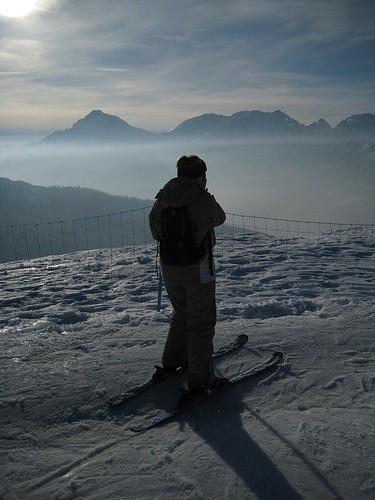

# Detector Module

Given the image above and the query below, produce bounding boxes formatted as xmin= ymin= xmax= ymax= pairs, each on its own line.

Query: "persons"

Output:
xmin=149 ymin=155 xmax=229 ymax=390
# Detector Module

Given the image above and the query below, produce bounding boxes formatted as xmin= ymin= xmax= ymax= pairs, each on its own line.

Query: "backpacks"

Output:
xmin=160 ymin=187 xmax=213 ymax=275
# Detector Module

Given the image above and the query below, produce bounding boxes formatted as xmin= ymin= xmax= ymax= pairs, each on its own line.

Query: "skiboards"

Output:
xmin=105 ymin=333 xmax=284 ymax=433
